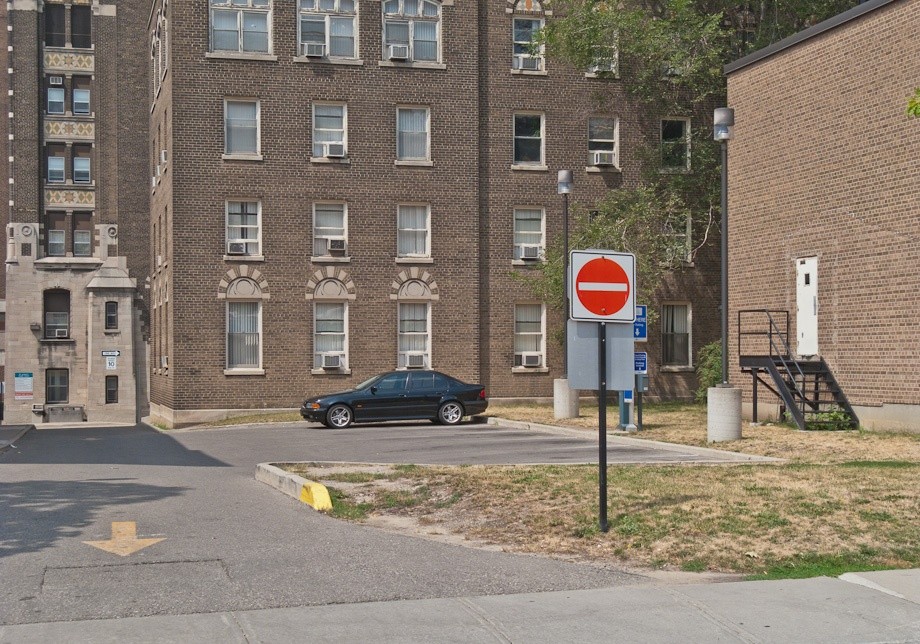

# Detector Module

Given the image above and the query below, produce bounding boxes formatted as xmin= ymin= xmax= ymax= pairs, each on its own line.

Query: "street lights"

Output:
xmin=553 ymin=168 xmax=581 ymax=421
xmin=707 ymin=108 xmax=744 ymax=440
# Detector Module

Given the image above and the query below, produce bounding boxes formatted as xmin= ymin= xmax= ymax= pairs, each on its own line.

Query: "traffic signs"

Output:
xmin=102 ymin=350 xmax=120 ymax=356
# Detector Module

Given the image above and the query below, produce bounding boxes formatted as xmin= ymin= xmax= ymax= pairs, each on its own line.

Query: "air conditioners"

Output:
xmin=405 ymin=353 xmax=425 ymax=367
xmin=387 ymin=45 xmax=409 ymax=60
xmin=594 ymin=57 xmax=612 ymax=72
xmin=522 ymin=352 xmax=541 ymax=366
xmin=301 ymin=43 xmax=325 ymax=56
xmin=323 ymin=142 xmax=345 ymax=158
xmin=588 ymin=151 xmax=615 ymax=166
xmin=327 ymin=239 xmax=345 ymax=251
xmin=518 ymin=56 xmax=539 ymax=70
xmin=56 ymin=329 xmax=67 ymax=337
xmin=518 ymin=246 xmax=539 ymax=258
xmin=322 ymin=354 xmax=341 ymax=367
xmin=228 ymin=242 xmax=247 ymax=253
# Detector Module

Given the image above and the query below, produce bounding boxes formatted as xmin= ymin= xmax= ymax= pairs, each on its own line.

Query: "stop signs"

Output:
xmin=576 ymin=258 xmax=629 ymax=315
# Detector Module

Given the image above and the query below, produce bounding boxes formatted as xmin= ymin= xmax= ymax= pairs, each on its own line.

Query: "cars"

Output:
xmin=301 ymin=370 xmax=488 ymax=429
